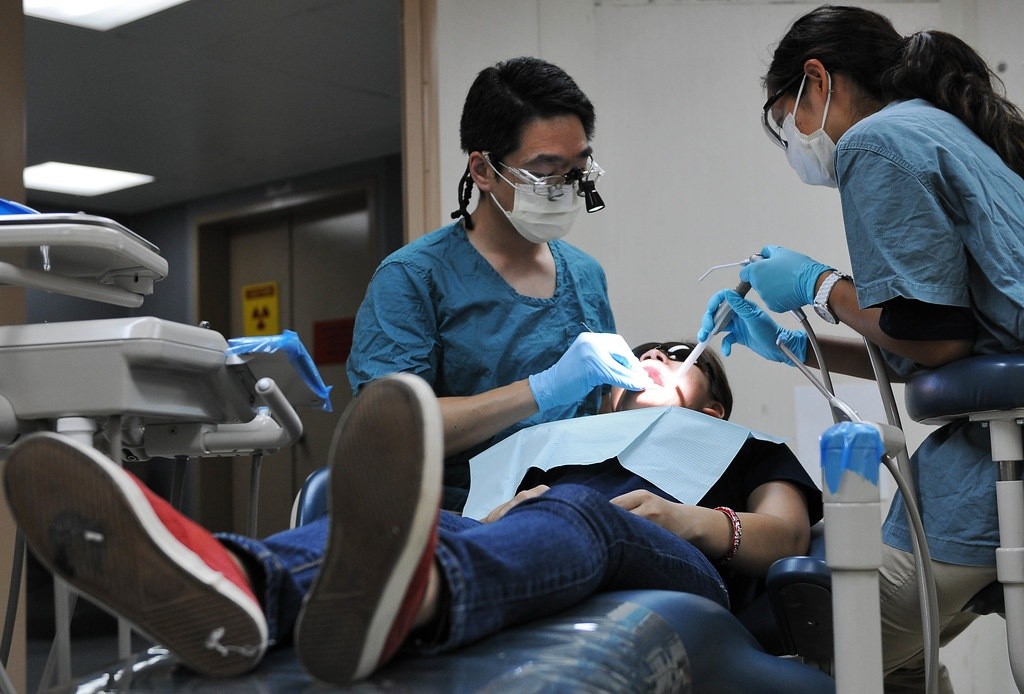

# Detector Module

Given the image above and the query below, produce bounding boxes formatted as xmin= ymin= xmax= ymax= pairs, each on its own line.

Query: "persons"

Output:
xmin=698 ymin=4 xmax=1024 ymax=694
xmin=345 ymin=59 xmax=652 ymax=497
xmin=0 ymin=343 xmax=826 ymax=683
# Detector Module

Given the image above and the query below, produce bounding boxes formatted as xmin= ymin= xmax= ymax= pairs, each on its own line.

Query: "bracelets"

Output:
xmin=711 ymin=507 xmax=741 ymax=569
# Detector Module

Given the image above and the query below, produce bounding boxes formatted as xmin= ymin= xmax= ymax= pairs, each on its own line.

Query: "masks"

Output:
xmin=480 ymin=150 xmax=586 ymax=245
xmin=776 ymin=70 xmax=839 ymax=189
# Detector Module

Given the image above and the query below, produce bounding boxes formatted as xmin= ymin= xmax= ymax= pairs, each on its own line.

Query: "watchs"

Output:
xmin=813 ymin=270 xmax=854 ymax=325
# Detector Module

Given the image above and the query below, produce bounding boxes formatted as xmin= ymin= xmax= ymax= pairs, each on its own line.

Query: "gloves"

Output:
xmin=697 ymin=289 xmax=806 ymax=367
xmin=739 ymin=245 xmax=839 ymax=314
xmin=528 ymin=332 xmax=653 ymax=412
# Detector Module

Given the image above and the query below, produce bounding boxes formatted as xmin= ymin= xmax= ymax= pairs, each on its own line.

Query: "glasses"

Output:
xmin=492 ymin=156 xmax=605 ymax=198
xmin=759 ymin=70 xmax=806 ymax=152
xmin=631 ymin=341 xmax=712 ymax=372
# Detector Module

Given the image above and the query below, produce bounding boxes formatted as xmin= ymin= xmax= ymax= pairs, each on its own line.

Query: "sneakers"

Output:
xmin=0 ymin=431 xmax=270 ymax=681
xmin=293 ymin=369 xmax=445 ymax=685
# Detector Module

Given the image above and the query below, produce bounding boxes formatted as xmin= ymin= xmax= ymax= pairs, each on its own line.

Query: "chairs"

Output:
xmin=57 ymin=465 xmax=836 ymax=694
